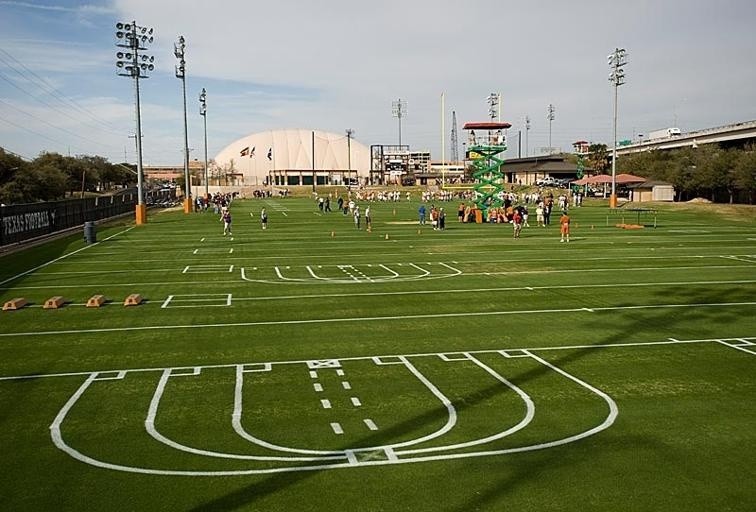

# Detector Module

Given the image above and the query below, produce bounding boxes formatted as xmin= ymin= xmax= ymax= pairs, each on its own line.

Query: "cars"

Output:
xmin=587 ymin=185 xmax=601 ymax=193
xmin=536 ymin=177 xmax=575 ymax=188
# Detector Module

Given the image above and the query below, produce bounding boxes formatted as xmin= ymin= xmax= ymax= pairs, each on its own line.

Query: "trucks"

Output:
xmin=648 ymin=126 xmax=681 ymax=142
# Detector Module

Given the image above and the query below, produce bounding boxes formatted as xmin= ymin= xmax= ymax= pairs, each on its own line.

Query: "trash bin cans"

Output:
xmin=83 ymin=221 xmax=97 ymax=246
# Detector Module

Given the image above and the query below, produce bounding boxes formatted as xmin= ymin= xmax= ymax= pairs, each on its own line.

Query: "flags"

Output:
xmin=249 ymin=147 xmax=255 ymax=159
xmin=239 ymin=147 xmax=248 ymax=157
xmin=267 ymin=147 xmax=271 ymax=160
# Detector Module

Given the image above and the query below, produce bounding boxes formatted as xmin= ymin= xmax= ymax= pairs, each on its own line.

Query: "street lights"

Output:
xmin=173 ymin=35 xmax=210 ymax=213
xmin=485 ymin=92 xmax=498 ymax=191
xmin=344 ymin=128 xmax=355 ymax=183
xmin=113 ymin=20 xmax=155 ymax=225
xmin=391 ymin=97 xmax=409 ymax=151
xmin=546 ymin=103 xmax=555 ymax=154
xmin=606 ymin=46 xmax=628 ymax=208
